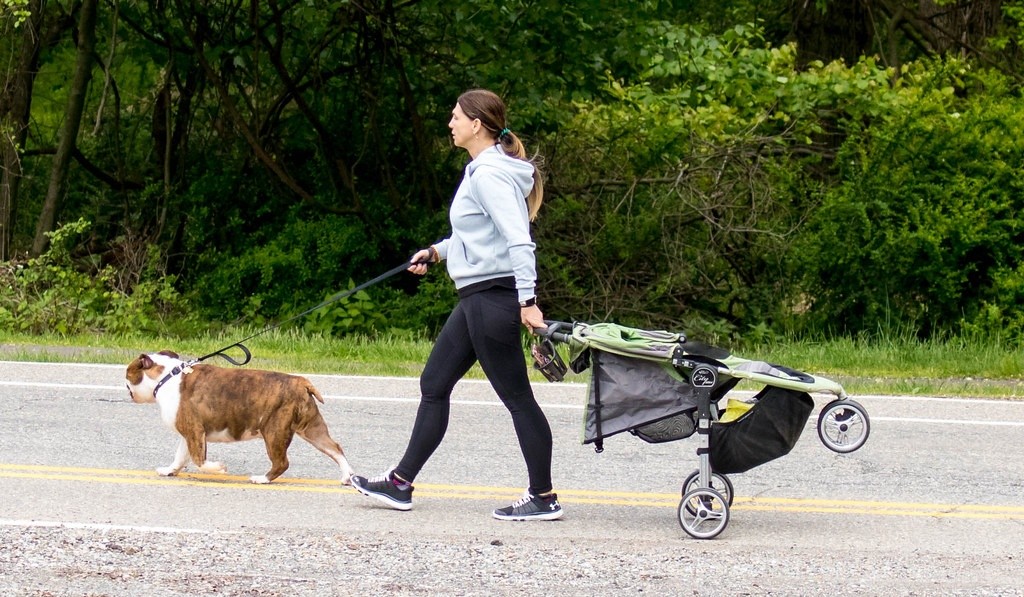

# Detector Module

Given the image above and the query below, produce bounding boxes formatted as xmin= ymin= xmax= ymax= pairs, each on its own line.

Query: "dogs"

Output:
xmin=124 ymin=349 xmax=359 ymax=485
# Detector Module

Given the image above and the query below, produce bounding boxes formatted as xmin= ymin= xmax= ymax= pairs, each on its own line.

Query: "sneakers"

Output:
xmin=350 ymin=470 xmax=415 ymax=510
xmin=492 ymin=486 xmax=564 ymax=521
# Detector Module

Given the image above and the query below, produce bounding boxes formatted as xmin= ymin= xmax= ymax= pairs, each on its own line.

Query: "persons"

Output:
xmin=350 ymin=90 xmax=564 ymax=521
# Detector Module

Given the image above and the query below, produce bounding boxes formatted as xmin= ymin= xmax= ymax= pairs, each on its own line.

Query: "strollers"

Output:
xmin=533 ymin=319 xmax=872 ymax=540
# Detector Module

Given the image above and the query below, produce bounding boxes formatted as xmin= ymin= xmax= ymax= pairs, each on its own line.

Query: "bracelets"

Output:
xmin=431 ymin=247 xmax=441 ymax=264
xmin=519 ymin=296 xmax=536 ymax=307
